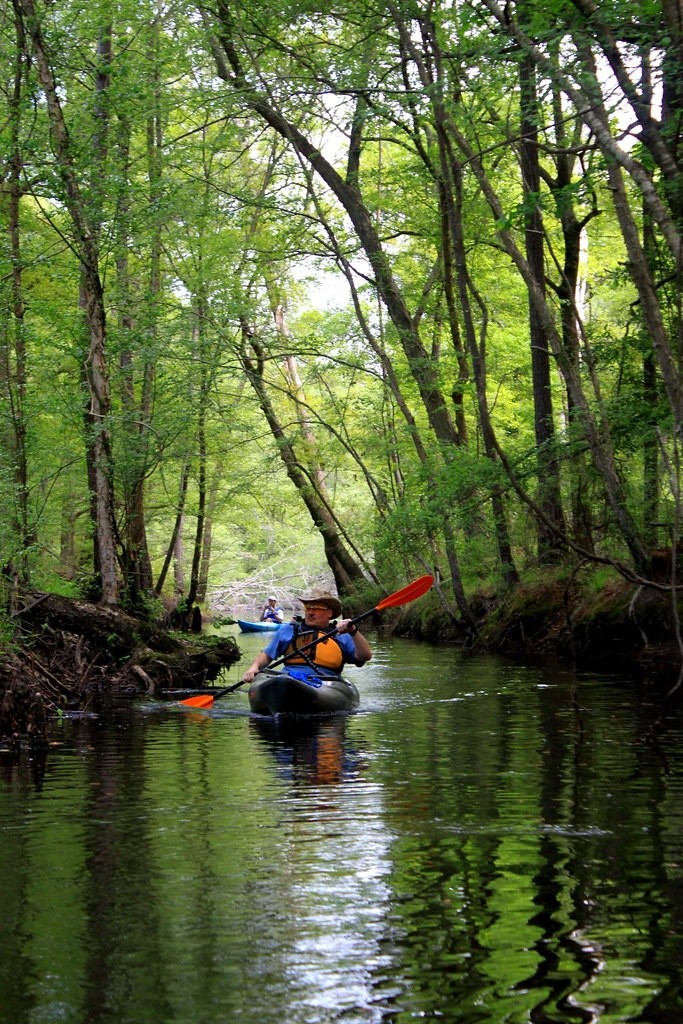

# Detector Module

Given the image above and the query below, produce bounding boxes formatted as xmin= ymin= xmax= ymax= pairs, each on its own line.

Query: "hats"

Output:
xmin=268 ymin=595 xmax=276 ymax=600
xmin=297 ymin=585 xmax=342 ymax=619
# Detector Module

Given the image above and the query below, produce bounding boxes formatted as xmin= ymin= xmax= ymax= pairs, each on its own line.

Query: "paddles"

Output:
xmin=179 ymin=574 xmax=434 ymax=710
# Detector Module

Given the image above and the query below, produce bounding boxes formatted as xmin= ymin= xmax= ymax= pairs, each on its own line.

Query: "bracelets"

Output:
xmin=350 ymin=627 xmax=358 ymax=636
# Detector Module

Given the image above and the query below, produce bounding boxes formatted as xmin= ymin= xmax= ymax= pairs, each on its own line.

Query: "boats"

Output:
xmin=248 ymin=669 xmax=361 ymax=717
xmin=237 ymin=618 xmax=288 ymax=633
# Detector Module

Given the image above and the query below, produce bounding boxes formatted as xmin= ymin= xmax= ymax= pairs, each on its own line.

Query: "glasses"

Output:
xmin=303 ymin=603 xmax=328 ymax=612
xmin=269 ymin=600 xmax=274 ymax=602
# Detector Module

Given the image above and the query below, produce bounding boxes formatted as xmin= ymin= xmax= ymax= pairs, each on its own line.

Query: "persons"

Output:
xmin=243 ymin=589 xmax=372 ymax=683
xmin=260 ymin=596 xmax=283 ymax=624
